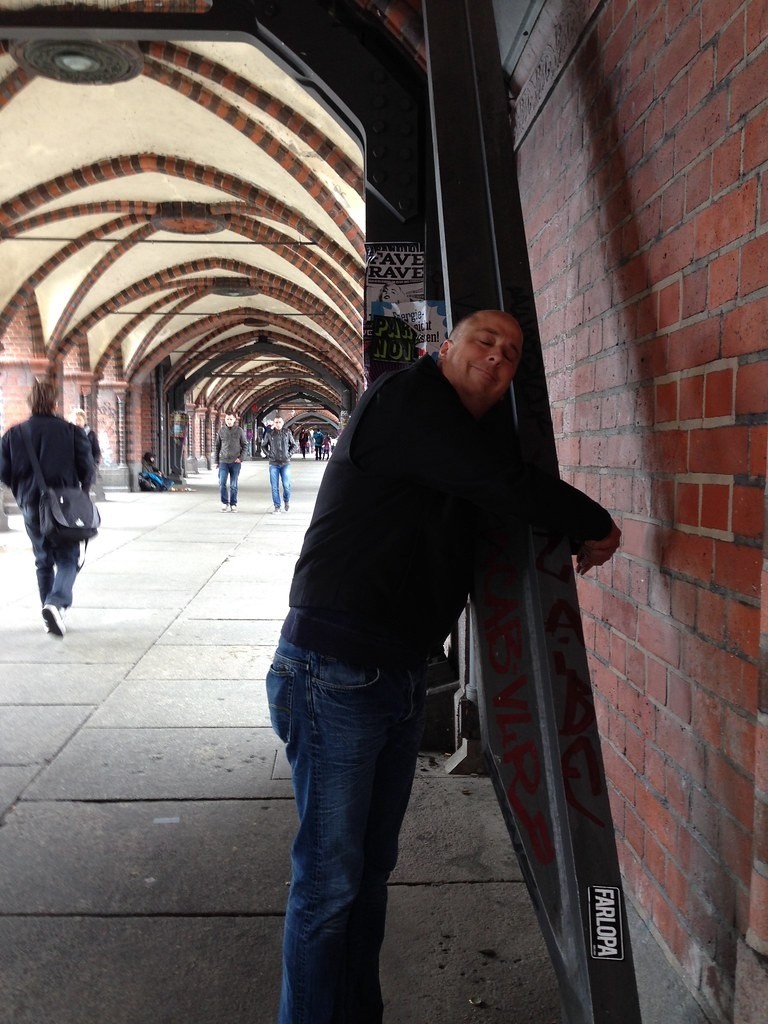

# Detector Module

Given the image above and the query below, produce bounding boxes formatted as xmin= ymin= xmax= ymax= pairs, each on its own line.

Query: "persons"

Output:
xmin=142 ymin=452 xmax=174 ymax=489
xmin=261 ymin=417 xmax=296 ymax=513
xmin=266 ymin=310 xmax=620 ymax=1024
xmin=0 ymin=382 xmax=100 ymax=638
xmin=214 ymin=412 xmax=247 ymax=513
xmin=300 ymin=428 xmax=336 ymax=461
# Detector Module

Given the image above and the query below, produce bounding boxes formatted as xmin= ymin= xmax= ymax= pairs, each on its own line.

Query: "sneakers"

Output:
xmin=43 ymin=605 xmax=65 ymax=636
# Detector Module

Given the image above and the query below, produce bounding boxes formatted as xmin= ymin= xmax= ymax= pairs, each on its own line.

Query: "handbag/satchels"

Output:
xmin=39 ymin=487 xmax=101 ymax=541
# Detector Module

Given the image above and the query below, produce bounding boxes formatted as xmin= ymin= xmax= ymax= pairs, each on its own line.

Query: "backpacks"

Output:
xmin=316 ymin=435 xmax=322 ymax=445
xmin=138 ymin=473 xmax=164 ymax=491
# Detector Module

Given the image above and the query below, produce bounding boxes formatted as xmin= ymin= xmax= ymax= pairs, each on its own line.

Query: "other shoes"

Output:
xmin=285 ymin=501 xmax=290 ymax=511
xmin=222 ymin=503 xmax=229 ymax=512
xmin=168 ymin=486 xmax=177 ymax=492
xmin=231 ymin=505 xmax=238 ymax=512
xmin=273 ymin=506 xmax=281 ymax=515
